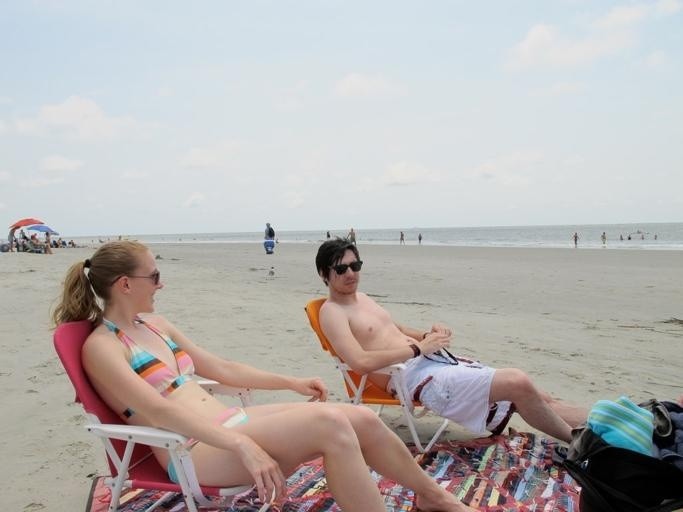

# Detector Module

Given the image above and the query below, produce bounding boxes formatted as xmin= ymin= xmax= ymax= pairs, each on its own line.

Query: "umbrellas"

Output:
xmin=8 ymin=217 xmax=45 ymax=230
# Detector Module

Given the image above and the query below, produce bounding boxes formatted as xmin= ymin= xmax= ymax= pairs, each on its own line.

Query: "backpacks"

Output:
xmin=551 ymin=398 xmax=682 ymax=512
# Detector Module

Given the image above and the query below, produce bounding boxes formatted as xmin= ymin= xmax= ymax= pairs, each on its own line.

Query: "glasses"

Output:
xmin=327 ymin=261 xmax=362 ymax=275
xmin=110 ymin=271 xmax=160 ymax=286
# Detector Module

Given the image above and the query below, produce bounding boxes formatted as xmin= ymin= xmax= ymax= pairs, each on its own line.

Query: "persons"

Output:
xmin=48 ymin=238 xmax=483 ymax=512
xmin=399 ymin=231 xmax=406 ymax=246
xmin=4 ymin=224 xmax=78 ymax=254
xmin=569 ymin=232 xmax=580 ymax=248
xmin=325 ymin=231 xmax=330 ymax=238
xmin=313 ymin=236 xmax=592 ymax=445
xmin=600 ymin=231 xmax=606 ymax=245
xmin=345 ymin=228 xmax=355 ymax=246
xmin=263 ymin=222 xmax=275 ymax=240
xmin=417 ymin=233 xmax=422 ymax=244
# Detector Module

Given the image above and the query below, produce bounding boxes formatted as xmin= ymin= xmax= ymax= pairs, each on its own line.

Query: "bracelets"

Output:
xmin=421 ymin=331 xmax=431 ymax=340
xmin=409 ymin=342 xmax=421 ymax=357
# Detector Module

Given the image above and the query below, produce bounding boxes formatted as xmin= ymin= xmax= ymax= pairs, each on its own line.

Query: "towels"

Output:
xmin=585 ymin=395 xmax=660 ymax=458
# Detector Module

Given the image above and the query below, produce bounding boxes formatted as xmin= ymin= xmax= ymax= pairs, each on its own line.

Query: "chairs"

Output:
xmin=305 ymin=297 xmax=449 ymax=453
xmin=53 ymin=318 xmax=275 ymax=511
